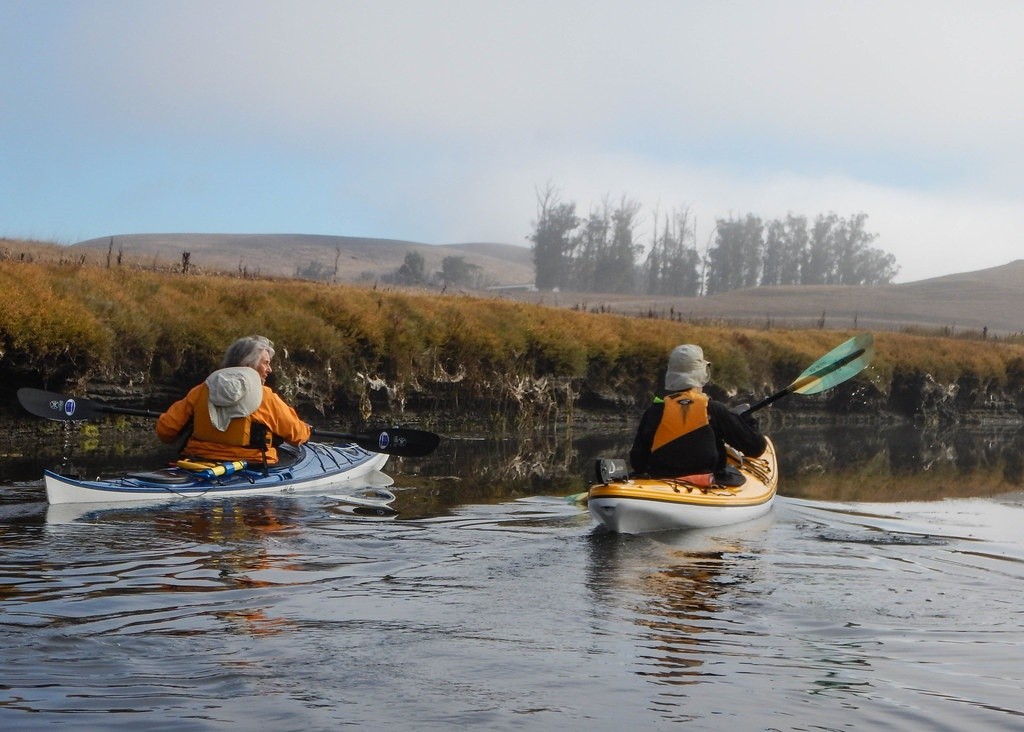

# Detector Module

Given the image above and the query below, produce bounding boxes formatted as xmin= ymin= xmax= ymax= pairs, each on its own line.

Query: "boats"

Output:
xmin=43 ymin=438 xmax=390 ymax=507
xmin=581 ymin=434 xmax=779 ymax=537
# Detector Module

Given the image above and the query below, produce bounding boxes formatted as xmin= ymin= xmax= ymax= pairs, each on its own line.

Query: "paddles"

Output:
xmin=17 ymin=387 xmax=441 ymax=459
xmin=575 ymin=330 xmax=876 ymax=501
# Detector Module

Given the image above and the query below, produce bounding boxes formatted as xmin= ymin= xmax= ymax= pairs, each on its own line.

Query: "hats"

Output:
xmin=205 ymin=366 xmax=263 ymax=431
xmin=665 ymin=343 xmax=711 ymax=391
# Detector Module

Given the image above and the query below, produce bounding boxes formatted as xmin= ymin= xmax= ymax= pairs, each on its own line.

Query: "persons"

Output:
xmin=156 ymin=335 xmax=311 ymax=465
xmin=629 ymin=345 xmax=768 ymax=484
